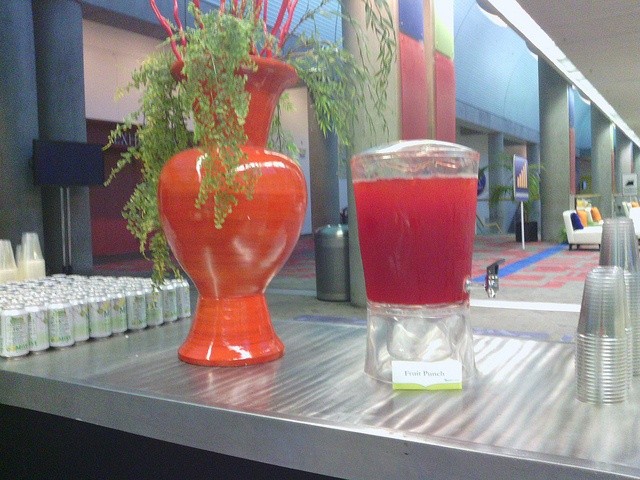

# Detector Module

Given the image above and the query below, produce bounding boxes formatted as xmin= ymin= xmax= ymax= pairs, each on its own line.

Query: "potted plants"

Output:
xmin=491 ymin=154 xmax=542 ymax=242
xmin=103 ymin=0 xmax=398 ymax=367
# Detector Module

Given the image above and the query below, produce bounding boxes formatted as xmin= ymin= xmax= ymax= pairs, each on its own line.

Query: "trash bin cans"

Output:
xmin=315 ymin=224 xmax=349 ymax=302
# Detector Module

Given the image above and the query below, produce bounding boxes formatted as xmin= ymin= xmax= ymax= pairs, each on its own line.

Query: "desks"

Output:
xmin=0 ymin=322 xmax=639 ymax=479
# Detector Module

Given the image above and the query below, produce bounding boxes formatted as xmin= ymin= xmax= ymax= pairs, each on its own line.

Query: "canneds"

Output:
xmin=0 ymin=273 xmax=190 ymax=358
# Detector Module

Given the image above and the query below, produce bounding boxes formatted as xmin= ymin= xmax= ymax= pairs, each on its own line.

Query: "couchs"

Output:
xmin=563 ymin=207 xmax=604 ymax=250
xmin=622 ymin=202 xmax=640 ymax=239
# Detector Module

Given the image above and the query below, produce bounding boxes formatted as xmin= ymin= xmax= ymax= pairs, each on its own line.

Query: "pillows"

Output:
xmin=571 ymin=213 xmax=583 ymax=229
xmin=627 ymin=203 xmax=632 ymax=212
xmin=592 ymin=207 xmax=601 ymax=221
xmin=577 ymin=211 xmax=587 ymax=226
xmin=586 ymin=213 xmax=593 ymax=226
xmin=632 ymin=202 xmax=639 ymax=207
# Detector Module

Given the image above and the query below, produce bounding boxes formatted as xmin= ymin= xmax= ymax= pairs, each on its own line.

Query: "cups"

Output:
xmin=351 ymin=139 xmax=480 ymax=385
xmin=0 ymin=231 xmax=47 ymax=285
xmin=575 ymin=266 xmax=633 ymax=405
xmin=598 ymin=216 xmax=640 ymax=377
